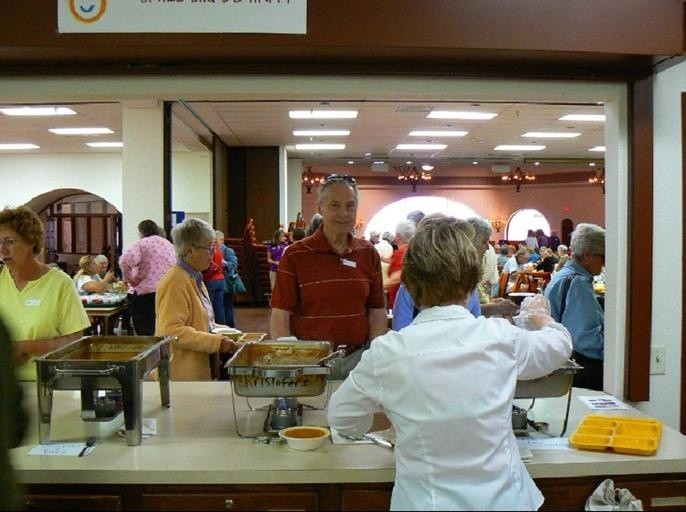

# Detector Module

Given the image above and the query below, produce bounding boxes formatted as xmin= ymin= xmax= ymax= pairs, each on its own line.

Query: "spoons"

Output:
xmin=79 ymin=436 xmax=96 ymax=456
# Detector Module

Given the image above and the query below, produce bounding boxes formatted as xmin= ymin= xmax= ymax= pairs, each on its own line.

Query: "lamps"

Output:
xmin=502 ymin=167 xmax=536 ymax=193
xmin=492 ymin=219 xmax=506 ymax=233
xmin=301 ymin=166 xmax=328 ymax=194
xmin=585 ymin=167 xmax=605 ymax=193
xmin=398 ymin=163 xmax=433 ymax=193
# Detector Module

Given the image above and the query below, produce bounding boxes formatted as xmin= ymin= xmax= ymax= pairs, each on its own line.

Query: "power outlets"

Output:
xmin=651 ymin=346 xmax=667 ymax=374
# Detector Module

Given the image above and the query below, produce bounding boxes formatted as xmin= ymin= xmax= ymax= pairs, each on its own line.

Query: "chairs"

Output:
xmin=497 ymin=273 xmax=510 ymax=299
xmin=223 ymin=217 xmax=278 ymax=308
xmin=528 ymin=271 xmax=551 ymax=293
xmin=512 ymin=273 xmax=524 ymax=293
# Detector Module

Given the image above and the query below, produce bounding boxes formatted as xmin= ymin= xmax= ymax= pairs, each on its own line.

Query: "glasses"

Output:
xmin=0 ymin=239 xmax=23 ymax=246
xmin=324 ymin=173 xmax=357 ymax=185
xmin=199 ymin=243 xmax=213 ymax=256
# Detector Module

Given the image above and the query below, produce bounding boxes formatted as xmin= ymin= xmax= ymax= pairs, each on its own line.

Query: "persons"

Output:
xmin=265 ymin=175 xmax=606 ymax=392
xmin=72 ymin=220 xmax=239 ymax=382
xmin=0 ymin=205 xmax=91 ymax=381
xmin=325 ymin=224 xmax=573 ymax=511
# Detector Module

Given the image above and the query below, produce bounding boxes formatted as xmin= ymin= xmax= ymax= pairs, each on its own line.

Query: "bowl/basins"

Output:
xmin=278 ymin=425 xmax=331 ymax=450
xmin=212 ymin=328 xmax=241 ymax=341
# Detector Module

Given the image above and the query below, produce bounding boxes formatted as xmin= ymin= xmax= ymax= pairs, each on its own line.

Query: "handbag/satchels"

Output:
xmin=230 ymin=273 xmax=247 ymax=295
xmin=569 ymin=352 xmax=606 ymax=392
